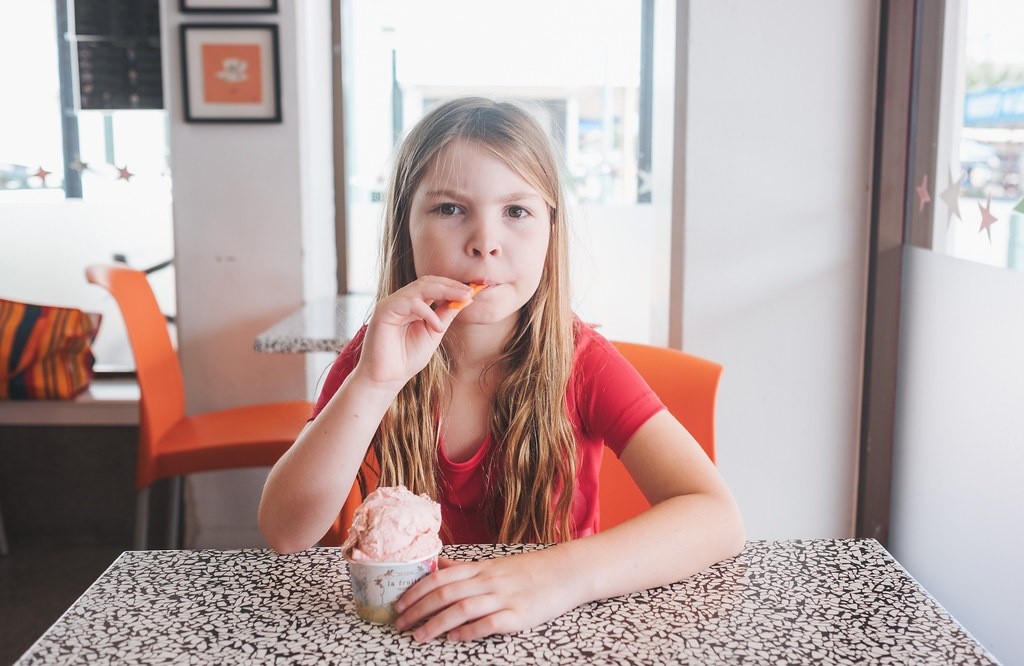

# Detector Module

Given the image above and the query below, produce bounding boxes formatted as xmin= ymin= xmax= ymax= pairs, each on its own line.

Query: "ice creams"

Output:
xmin=342 ymin=486 xmax=442 ymax=624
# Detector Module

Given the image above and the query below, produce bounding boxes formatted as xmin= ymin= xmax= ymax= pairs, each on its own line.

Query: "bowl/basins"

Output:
xmin=346 ymin=538 xmax=443 ymax=625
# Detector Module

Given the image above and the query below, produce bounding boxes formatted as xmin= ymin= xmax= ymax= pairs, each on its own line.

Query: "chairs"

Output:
xmin=83 ymin=263 xmax=314 ymax=551
xmin=595 ymin=341 xmax=722 ymax=532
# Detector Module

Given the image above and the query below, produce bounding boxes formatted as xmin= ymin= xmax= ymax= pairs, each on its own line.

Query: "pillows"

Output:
xmin=0 ymin=299 xmax=102 ymax=401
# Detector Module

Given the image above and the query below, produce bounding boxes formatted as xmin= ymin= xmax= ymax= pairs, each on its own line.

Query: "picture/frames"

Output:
xmin=179 ymin=0 xmax=278 ymax=13
xmin=179 ymin=23 xmax=283 ymax=124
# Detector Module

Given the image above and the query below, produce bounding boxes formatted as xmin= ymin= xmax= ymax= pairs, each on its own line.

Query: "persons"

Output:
xmin=260 ymin=98 xmax=747 ymax=644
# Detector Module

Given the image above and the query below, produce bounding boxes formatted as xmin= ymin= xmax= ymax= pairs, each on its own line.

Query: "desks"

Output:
xmin=250 ymin=293 xmax=378 ymax=353
xmin=10 ymin=538 xmax=1004 ymax=666
xmin=1 ymin=376 xmax=143 ymax=613
